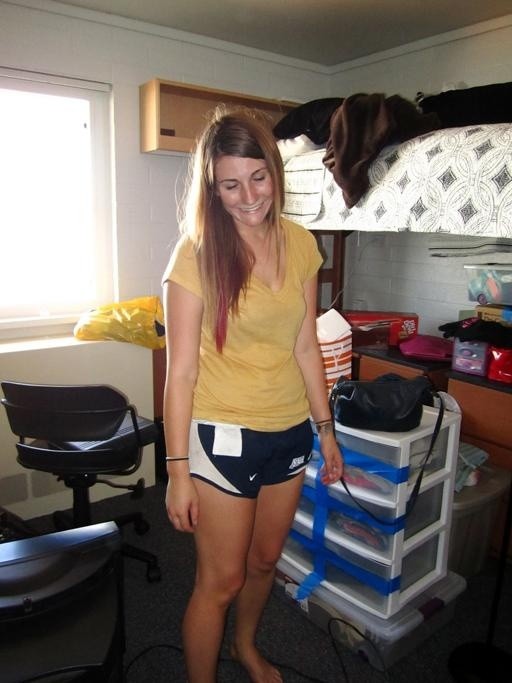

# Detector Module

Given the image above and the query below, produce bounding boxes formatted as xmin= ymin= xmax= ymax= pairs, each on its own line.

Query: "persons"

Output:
xmin=159 ymin=106 xmax=344 ymax=683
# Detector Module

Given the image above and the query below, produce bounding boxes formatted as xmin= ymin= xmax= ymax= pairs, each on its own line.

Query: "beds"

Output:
xmin=271 ymin=123 xmax=511 ymax=240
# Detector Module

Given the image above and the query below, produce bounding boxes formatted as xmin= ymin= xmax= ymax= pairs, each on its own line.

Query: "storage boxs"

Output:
xmin=272 ymin=558 xmax=468 ymax=673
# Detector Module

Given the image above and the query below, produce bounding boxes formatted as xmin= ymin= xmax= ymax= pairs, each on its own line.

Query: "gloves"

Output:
xmin=440 ymin=317 xmax=512 ymax=347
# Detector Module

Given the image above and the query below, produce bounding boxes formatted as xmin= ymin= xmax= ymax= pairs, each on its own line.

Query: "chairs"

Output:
xmin=0 ymin=379 xmax=164 ymax=582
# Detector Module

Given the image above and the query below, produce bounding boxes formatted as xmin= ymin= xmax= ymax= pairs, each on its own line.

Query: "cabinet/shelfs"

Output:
xmin=137 ymin=76 xmax=297 ymax=159
xmin=445 ymin=378 xmax=511 ymax=555
xmin=274 ymin=408 xmax=461 ymax=619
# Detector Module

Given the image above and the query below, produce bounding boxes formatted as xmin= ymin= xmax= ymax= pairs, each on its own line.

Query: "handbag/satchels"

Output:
xmin=318 ymin=332 xmax=354 ymax=396
xmin=327 ymin=371 xmax=444 ymax=527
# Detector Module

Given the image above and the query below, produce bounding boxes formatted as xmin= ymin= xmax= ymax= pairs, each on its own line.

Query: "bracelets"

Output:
xmin=165 ymin=456 xmax=189 ymax=461
xmin=315 ymin=419 xmax=336 ymax=427
